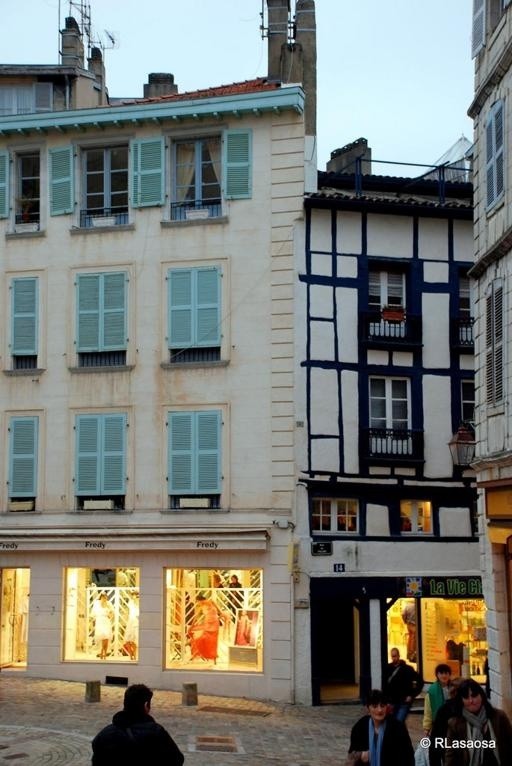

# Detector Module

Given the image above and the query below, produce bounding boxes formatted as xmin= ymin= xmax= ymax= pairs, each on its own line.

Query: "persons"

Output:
xmin=348 ymin=689 xmax=415 ymax=766
xmin=429 ymin=677 xmax=465 ymax=766
xmin=383 ymin=648 xmax=425 ymax=722
xmin=118 ymin=592 xmax=140 ymax=661
xmin=401 ymin=598 xmax=417 ymax=663
xmin=443 ymin=679 xmax=512 ymax=766
xmin=423 ymin=664 xmax=455 ymax=737
xmin=91 ymin=684 xmax=184 ymax=766
xmin=90 ymin=592 xmax=115 ymax=659
xmin=188 ymin=595 xmax=230 ymax=665
xmin=483 ymin=651 xmax=491 ymax=700
xmin=228 ymin=575 xmax=244 ymax=623
xmin=212 ymin=575 xmax=224 ymax=588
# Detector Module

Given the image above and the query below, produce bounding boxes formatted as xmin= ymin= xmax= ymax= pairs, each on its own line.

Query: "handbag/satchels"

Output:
xmin=414 ymin=736 xmax=429 ymax=766
